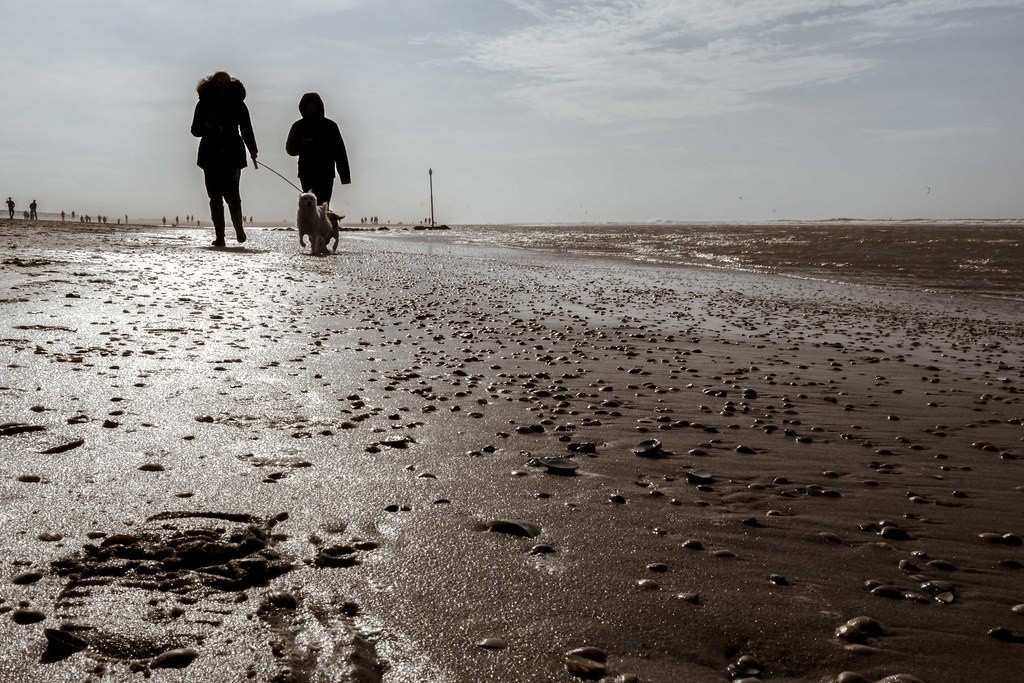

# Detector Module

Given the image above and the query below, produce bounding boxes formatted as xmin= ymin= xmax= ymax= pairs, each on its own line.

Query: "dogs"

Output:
xmin=297 ymin=189 xmax=345 ymax=256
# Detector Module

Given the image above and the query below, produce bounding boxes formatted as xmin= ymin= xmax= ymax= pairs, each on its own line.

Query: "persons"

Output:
xmin=5 ymin=196 xmax=433 ymax=229
xmin=190 ymin=72 xmax=258 ymax=247
xmin=286 ymin=93 xmax=350 ymax=255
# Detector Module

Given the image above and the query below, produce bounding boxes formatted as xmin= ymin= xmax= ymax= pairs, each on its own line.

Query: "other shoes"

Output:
xmin=211 ymin=240 xmax=225 ymax=246
xmin=236 ymin=227 xmax=246 ymax=243
